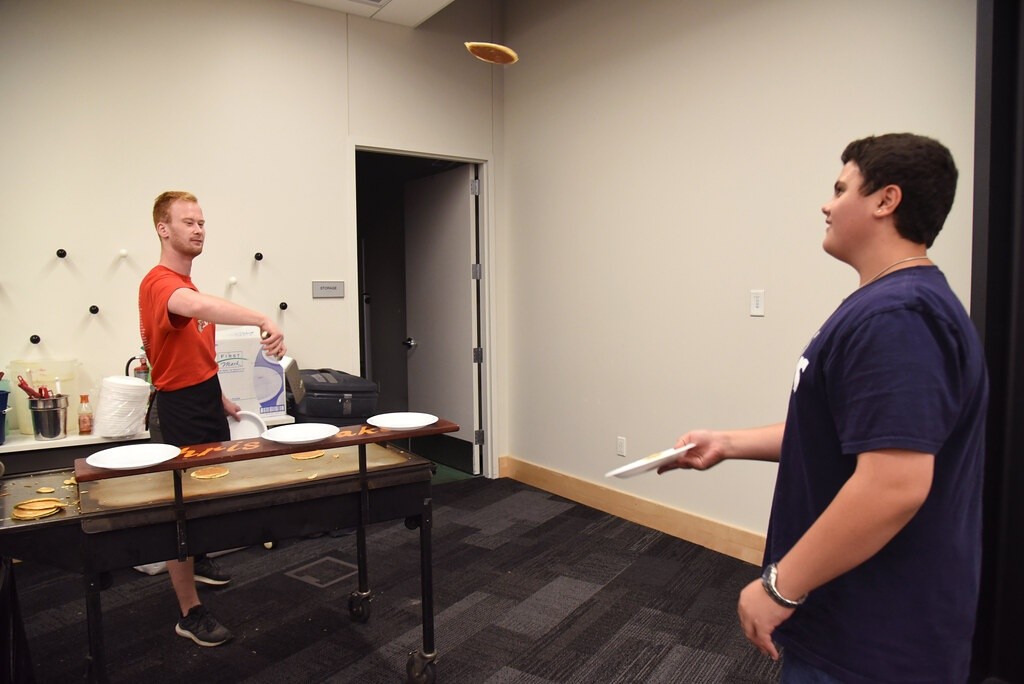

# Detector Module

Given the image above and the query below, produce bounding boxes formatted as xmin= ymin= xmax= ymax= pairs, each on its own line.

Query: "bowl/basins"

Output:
xmin=228 ymin=409 xmax=268 ymax=441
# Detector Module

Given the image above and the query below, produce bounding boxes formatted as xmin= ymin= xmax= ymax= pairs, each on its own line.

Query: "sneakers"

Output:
xmin=192 ymin=555 xmax=231 ymax=585
xmin=174 ymin=604 xmax=233 ymax=646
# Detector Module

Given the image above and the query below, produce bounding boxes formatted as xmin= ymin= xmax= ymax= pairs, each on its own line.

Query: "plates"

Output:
xmin=260 ymin=422 xmax=340 ymax=444
xmin=366 ymin=411 xmax=439 ymax=431
xmin=93 ymin=376 xmax=151 ymax=437
xmin=85 ymin=443 xmax=181 ymax=470
xmin=605 ymin=444 xmax=698 ymax=479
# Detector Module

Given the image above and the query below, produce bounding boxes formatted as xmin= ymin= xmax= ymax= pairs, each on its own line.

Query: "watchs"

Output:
xmin=762 ymin=563 xmax=808 ymax=607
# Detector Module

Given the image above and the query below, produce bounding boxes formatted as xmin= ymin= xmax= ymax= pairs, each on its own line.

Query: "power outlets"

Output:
xmin=616 ymin=437 xmax=628 ymax=458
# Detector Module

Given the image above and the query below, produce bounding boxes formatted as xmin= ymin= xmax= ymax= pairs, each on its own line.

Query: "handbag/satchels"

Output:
xmin=285 ymin=368 xmax=379 ymax=425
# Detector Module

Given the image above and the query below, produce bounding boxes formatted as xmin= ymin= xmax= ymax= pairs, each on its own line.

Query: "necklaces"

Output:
xmin=860 ymin=256 xmax=929 ymax=287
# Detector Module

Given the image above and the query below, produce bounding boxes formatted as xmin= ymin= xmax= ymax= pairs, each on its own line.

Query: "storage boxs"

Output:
xmin=215 ymin=325 xmax=287 ymax=417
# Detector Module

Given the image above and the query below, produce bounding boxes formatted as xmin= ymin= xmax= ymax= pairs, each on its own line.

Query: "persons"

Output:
xmin=139 ymin=192 xmax=286 ymax=647
xmin=657 ymin=132 xmax=989 ymax=684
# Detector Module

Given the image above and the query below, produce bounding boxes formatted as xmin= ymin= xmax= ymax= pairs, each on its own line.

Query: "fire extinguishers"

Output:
xmin=125 ymin=352 xmax=149 ymax=381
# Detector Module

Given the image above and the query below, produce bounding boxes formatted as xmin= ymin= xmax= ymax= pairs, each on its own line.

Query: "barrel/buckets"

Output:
xmin=0 ymin=389 xmax=13 ymax=446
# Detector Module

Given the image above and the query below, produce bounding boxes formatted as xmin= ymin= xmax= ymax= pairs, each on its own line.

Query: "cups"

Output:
xmin=26 ymin=395 xmax=69 ymax=440
xmin=15 ymin=387 xmax=34 ymax=436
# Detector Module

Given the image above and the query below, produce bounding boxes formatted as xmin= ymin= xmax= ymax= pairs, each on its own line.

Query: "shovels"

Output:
xmin=261 ymin=330 xmax=306 ymax=404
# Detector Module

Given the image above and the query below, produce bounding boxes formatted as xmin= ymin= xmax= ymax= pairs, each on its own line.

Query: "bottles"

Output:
xmin=78 ymin=394 xmax=92 ymax=436
